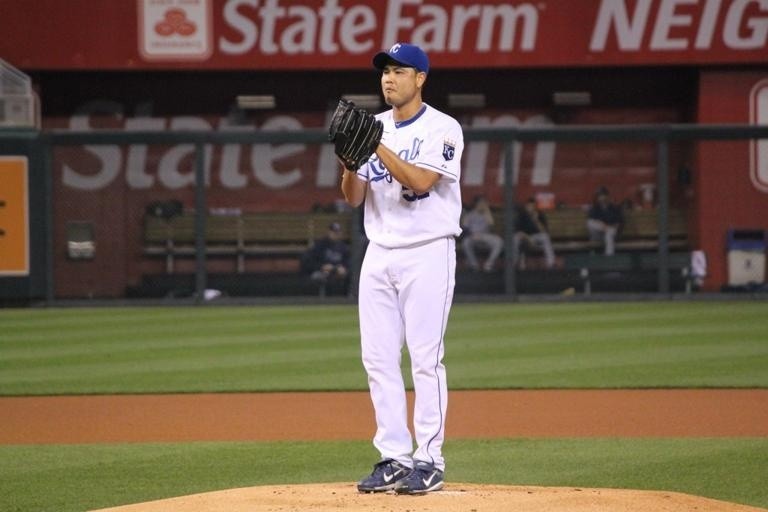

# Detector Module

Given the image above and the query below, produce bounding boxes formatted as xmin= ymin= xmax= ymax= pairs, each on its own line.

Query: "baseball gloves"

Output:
xmin=327 ymin=100 xmax=384 ymax=172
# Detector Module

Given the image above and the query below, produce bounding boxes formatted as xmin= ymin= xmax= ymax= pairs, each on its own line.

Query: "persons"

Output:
xmin=325 ymin=38 xmax=467 ymax=497
xmin=300 ymin=220 xmax=351 ymax=297
xmin=462 ymin=192 xmax=505 ymax=273
xmin=512 ymin=196 xmax=557 ymax=272
xmin=582 ymin=183 xmax=624 ymax=258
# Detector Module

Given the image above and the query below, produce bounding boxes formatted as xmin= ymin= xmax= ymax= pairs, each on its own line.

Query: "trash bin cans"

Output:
xmin=726 ymin=229 xmax=767 ymax=288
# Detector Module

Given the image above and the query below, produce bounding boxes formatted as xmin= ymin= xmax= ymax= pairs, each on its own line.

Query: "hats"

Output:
xmin=371 ymin=41 xmax=431 ymax=78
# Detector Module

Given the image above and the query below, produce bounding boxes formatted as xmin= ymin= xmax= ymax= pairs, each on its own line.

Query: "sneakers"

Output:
xmin=356 ymin=457 xmax=415 ymax=494
xmin=392 ymin=459 xmax=446 ymax=495
